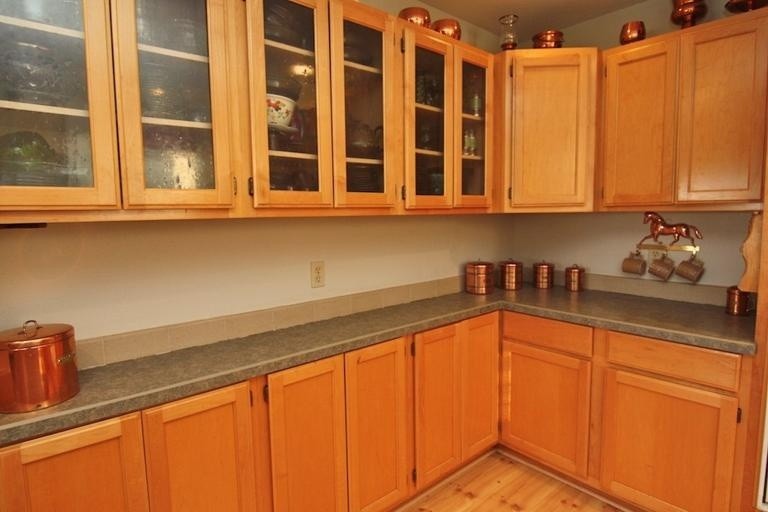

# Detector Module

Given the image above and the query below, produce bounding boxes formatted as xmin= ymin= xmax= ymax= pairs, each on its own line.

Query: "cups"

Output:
xmin=622 ymin=251 xmax=647 ymax=276
xmin=648 ymin=253 xmax=674 ymax=281
xmin=676 ymin=256 xmax=705 ymax=284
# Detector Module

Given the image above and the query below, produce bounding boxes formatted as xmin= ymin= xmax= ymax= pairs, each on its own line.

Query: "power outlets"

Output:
xmin=309 ymin=259 xmax=327 ymax=290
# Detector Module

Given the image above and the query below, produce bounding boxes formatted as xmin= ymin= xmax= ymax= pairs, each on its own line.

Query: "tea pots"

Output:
xmin=346 ymin=119 xmax=382 ymax=160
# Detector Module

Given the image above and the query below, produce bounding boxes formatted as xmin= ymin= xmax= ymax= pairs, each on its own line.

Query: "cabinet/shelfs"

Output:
xmin=241 ymin=0 xmax=399 ymax=221
xmin=0 ymin=356 xmax=261 ymax=510
xmin=0 ymin=2 xmax=241 ymax=230
xmin=501 ymin=303 xmax=595 ymax=480
xmin=411 ymin=301 xmax=501 ymax=504
xmin=260 ymin=326 xmax=412 ymax=512
xmin=596 ymin=326 xmax=747 ymax=512
xmin=602 ymin=3 xmax=767 ymax=214
xmin=398 ymin=15 xmax=499 ymax=220
xmin=498 ymin=43 xmax=604 ymax=215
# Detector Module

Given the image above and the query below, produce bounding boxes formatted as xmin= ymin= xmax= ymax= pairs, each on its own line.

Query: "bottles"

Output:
xmin=461 ymin=88 xmax=485 ymax=156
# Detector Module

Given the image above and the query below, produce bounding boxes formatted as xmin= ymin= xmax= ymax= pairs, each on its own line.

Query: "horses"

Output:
xmin=639 ymin=211 xmax=703 ymax=247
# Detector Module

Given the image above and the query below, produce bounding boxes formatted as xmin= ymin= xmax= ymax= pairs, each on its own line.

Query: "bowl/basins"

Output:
xmin=264 ymin=3 xmax=317 ymax=191
xmin=1 ymin=2 xmax=215 ymax=189
